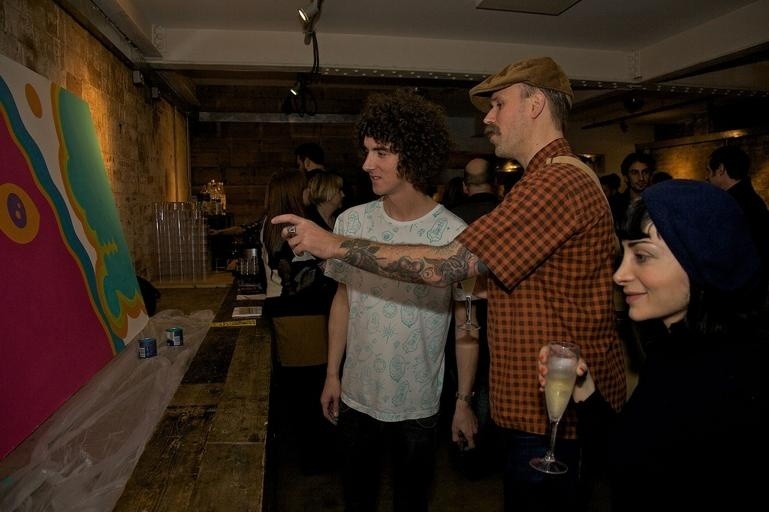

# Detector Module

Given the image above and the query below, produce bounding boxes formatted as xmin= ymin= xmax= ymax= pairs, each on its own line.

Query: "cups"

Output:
xmin=149 ymin=199 xmax=208 ymax=284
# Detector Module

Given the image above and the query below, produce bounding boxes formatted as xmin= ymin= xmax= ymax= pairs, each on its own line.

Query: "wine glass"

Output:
xmin=456 ymin=274 xmax=482 ymax=333
xmin=528 ymin=341 xmax=578 ymax=473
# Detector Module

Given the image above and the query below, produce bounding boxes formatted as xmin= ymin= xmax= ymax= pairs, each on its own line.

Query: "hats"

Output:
xmin=468 ymin=56 xmax=574 ymax=114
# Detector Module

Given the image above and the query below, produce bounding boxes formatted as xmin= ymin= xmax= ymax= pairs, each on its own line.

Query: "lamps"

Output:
xmin=289 ymin=80 xmax=301 ymax=96
xmin=298 ymin=0 xmax=321 ymax=24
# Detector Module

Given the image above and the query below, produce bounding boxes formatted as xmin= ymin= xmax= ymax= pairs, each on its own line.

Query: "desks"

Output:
xmin=0 ymin=270 xmax=273 ymax=512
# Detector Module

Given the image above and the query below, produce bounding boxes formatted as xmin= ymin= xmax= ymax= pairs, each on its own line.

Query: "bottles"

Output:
xmin=201 ymin=179 xmax=227 ymax=214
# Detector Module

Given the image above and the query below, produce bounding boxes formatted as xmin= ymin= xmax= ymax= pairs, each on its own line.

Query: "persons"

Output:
xmin=202 ymin=59 xmax=768 ymax=512
